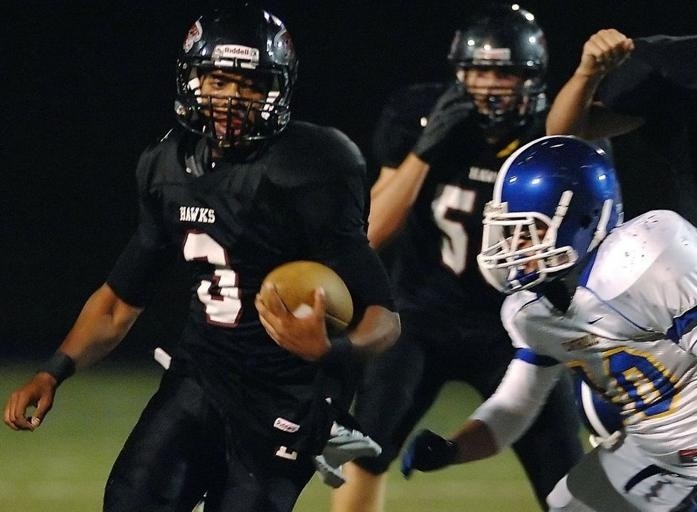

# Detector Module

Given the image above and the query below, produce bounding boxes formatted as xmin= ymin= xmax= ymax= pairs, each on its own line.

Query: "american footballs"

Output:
xmin=260 ymin=259 xmax=354 ymax=336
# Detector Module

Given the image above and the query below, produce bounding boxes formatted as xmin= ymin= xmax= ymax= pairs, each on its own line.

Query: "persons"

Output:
xmin=331 ymin=2 xmax=588 ymax=512
xmin=1 ymin=67 xmax=404 ymax=512
xmin=542 ymin=3 xmax=697 ymax=228
xmin=395 ymin=131 xmax=697 ymax=511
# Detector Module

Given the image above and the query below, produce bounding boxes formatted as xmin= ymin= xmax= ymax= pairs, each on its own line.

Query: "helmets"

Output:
xmin=478 ymin=135 xmax=625 ymax=296
xmin=173 ymin=7 xmax=298 ymax=150
xmin=446 ymin=3 xmax=547 ymax=127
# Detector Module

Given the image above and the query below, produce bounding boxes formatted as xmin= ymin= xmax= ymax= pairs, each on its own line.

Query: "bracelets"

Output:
xmin=325 ymin=335 xmax=371 ymax=371
xmin=35 ymin=347 xmax=78 ymax=387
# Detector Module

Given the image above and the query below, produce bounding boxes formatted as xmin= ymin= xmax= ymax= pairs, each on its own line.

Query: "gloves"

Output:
xmin=414 ymin=81 xmax=476 ymax=163
xmin=400 ymin=428 xmax=458 ymax=479
